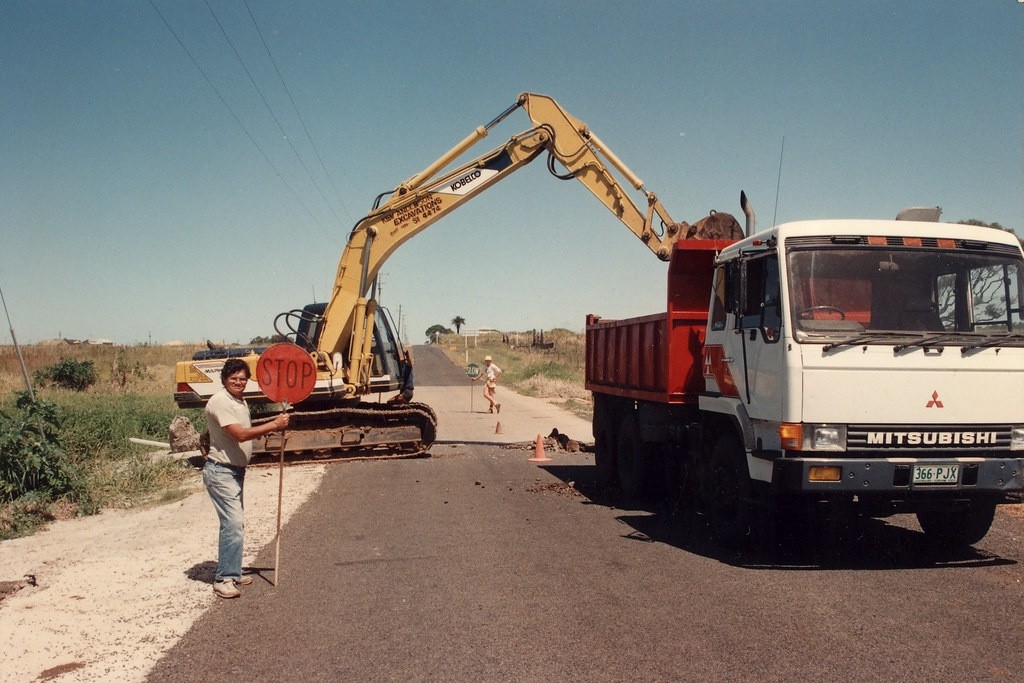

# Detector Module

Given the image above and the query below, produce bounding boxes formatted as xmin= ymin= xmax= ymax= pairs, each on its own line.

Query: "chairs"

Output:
xmin=898 ymin=296 xmax=947 ymax=332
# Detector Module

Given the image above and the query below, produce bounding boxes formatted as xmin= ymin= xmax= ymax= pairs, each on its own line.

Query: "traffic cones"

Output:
xmin=526 ymin=434 xmax=552 ymax=461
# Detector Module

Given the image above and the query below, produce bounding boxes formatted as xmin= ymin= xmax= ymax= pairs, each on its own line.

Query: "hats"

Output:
xmin=485 ymin=356 xmax=492 ymax=361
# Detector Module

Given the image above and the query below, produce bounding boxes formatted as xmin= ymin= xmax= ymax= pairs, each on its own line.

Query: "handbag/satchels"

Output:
xmin=487 ymin=381 xmax=496 ymax=388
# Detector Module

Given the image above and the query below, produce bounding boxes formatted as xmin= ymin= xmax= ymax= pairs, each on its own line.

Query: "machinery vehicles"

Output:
xmin=170 ymin=93 xmax=748 ymax=468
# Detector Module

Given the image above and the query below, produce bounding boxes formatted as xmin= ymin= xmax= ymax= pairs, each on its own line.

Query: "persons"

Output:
xmin=201 ymin=358 xmax=290 ymax=598
xmin=472 ymin=355 xmax=503 ymax=414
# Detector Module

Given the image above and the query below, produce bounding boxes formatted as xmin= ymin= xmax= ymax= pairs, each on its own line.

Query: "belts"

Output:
xmin=208 ymin=457 xmax=246 ymax=471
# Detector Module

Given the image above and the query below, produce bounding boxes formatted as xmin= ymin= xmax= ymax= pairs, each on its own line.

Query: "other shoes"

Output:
xmin=495 ymin=404 xmax=501 ymax=413
xmin=488 ymin=406 xmax=493 ymax=413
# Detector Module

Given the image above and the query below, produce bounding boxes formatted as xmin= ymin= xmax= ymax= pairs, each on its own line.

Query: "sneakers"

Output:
xmin=232 ymin=573 xmax=253 ymax=585
xmin=213 ymin=577 xmax=241 ymax=598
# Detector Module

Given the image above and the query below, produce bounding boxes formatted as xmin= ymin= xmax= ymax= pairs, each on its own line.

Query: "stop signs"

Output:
xmin=255 ymin=342 xmax=318 ymax=404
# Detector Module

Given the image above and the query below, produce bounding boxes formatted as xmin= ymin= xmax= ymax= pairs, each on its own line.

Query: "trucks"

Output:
xmin=581 ymin=191 xmax=1023 ymax=545
